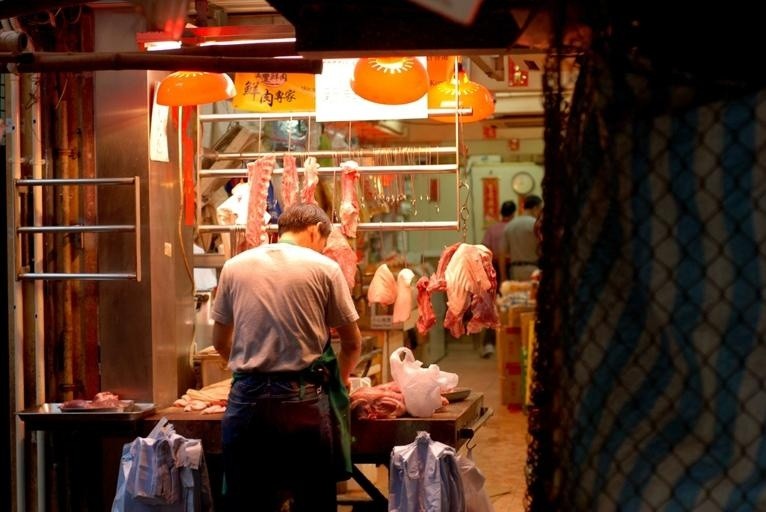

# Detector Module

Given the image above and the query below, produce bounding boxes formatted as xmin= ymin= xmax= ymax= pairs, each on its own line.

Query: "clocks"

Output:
xmin=511 ymin=172 xmax=535 ymax=195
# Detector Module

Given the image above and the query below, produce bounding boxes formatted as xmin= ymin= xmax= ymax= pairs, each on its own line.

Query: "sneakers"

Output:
xmin=480 ymin=343 xmax=495 ymax=360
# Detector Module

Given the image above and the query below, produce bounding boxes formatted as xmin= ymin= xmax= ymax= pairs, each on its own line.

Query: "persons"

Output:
xmin=499 ymin=194 xmax=542 ymax=281
xmin=479 ymin=200 xmax=516 ymax=358
xmin=208 ymin=203 xmax=362 ymax=511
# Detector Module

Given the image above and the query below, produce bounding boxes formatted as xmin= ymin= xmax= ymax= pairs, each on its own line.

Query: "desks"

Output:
xmin=142 ymin=388 xmax=496 ymax=512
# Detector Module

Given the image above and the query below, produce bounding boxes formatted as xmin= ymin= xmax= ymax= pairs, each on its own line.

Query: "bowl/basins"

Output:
xmin=441 ymin=386 xmax=473 ymax=401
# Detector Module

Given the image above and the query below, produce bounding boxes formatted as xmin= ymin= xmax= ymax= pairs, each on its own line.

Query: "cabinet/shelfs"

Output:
xmin=193 ymin=347 xmax=233 ymax=386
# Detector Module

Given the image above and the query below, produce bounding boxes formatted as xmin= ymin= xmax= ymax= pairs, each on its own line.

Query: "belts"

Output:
xmin=510 ymin=261 xmax=535 ymax=267
